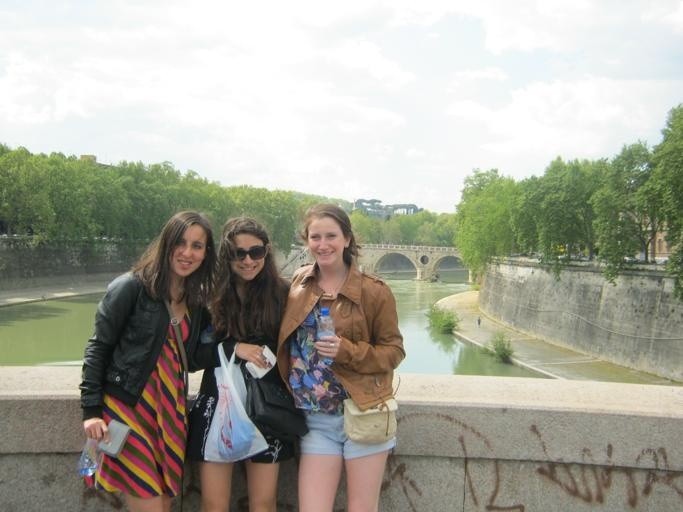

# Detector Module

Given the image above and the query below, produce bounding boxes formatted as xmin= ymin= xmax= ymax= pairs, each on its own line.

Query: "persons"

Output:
xmin=77 ymin=210 xmax=221 ymax=511
xmin=184 ymin=214 xmax=307 ymax=512
xmin=275 ymin=203 xmax=407 ymax=511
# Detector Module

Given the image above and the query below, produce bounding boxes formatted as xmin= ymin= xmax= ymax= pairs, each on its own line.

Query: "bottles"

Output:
xmin=79 ymin=438 xmax=101 ymax=478
xmin=317 ymin=308 xmax=335 ymax=365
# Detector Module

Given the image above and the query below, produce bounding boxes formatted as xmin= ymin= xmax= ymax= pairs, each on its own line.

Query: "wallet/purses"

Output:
xmin=99 ymin=420 xmax=130 ymax=458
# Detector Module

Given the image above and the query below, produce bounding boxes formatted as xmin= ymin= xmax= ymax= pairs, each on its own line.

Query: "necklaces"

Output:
xmin=331 ymin=269 xmax=347 ymax=296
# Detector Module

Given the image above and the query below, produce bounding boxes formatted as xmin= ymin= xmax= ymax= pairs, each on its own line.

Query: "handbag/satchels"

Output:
xmin=343 ymin=396 xmax=398 ymax=443
xmin=246 ymin=378 xmax=298 ymax=432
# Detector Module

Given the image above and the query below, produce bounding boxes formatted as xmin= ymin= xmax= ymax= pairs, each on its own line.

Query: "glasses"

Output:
xmin=227 ymin=243 xmax=266 ymax=260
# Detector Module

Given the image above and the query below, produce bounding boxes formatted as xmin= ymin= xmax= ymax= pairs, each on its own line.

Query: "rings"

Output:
xmin=329 ymin=343 xmax=334 ymax=348
xmin=255 ymin=355 xmax=258 ymax=359
